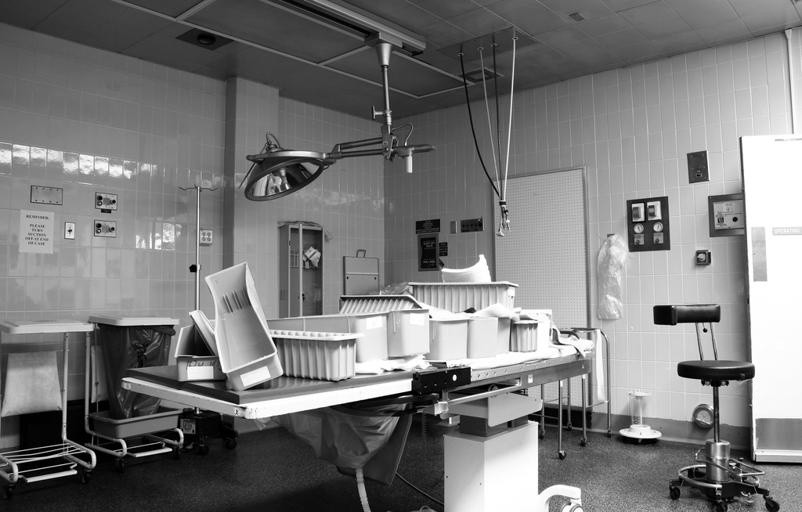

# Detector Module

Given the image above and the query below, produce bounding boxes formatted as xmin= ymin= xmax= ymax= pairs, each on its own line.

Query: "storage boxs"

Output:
xmin=204 ymin=262 xmax=283 ymax=393
xmin=338 ymin=294 xmax=423 ymax=312
xmin=427 ymin=317 xmax=470 ymax=361
xmin=269 ymin=328 xmax=361 ymax=382
xmin=468 ymin=318 xmax=500 ymax=358
xmin=175 ymin=322 xmax=225 ymax=383
xmin=267 ymin=312 xmax=389 ymax=365
xmin=406 ymin=281 xmax=519 ymax=351
xmin=387 ymin=309 xmax=430 ymax=357
xmin=511 ymin=320 xmax=551 ymax=352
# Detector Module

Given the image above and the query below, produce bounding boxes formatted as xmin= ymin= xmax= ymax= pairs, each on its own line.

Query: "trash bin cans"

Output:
xmin=89 ymin=317 xmax=179 ymax=419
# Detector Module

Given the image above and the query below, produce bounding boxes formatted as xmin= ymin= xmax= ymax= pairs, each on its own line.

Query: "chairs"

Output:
xmin=654 ymin=305 xmax=779 ymax=512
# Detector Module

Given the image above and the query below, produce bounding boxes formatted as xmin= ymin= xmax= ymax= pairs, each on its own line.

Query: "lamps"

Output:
xmin=238 ymin=32 xmax=435 ymax=202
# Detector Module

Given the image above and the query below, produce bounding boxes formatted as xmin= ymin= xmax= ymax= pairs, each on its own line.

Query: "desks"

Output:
xmin=119 ymin=340 xmax=594 ymax=511
